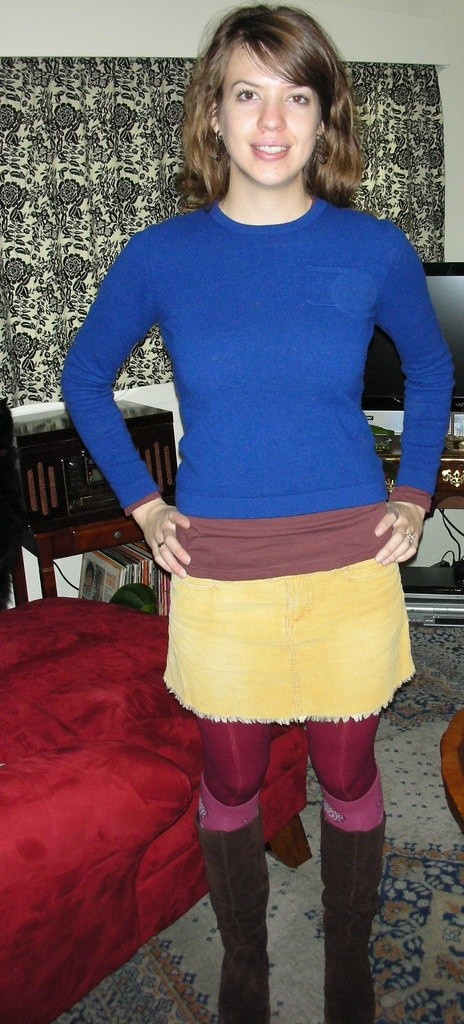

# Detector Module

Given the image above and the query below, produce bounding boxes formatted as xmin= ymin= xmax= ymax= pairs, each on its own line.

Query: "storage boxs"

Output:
xmin=19 ymin=403 xmax=179 ymax=533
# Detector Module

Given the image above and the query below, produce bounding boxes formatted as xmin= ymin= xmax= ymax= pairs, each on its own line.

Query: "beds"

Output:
xmin=1 ymin=595 xmax=312 ymax=1023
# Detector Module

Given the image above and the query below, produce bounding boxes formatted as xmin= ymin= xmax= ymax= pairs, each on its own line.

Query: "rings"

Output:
xmin=158 ymin=542 xmax=165 ymax=548
xmin=406 ymin=533 xmax=415 ymax=544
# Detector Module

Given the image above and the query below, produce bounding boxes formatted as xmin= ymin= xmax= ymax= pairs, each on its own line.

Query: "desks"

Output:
xmin=374 ymin=436 xmax=464 ymax=512
xmin=13 ymin=503 xmax=179 ymax=600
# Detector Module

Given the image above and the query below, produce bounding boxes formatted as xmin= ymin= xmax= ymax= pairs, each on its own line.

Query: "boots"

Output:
xmin=319 ymin=806 xmax=386 ymax=1024
xmin=195 ymin=803 xmax=270 ymax=1024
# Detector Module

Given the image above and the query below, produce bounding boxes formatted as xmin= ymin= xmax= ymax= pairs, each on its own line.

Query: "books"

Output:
xmin=78 ymin=540 xmax=170 ymax=617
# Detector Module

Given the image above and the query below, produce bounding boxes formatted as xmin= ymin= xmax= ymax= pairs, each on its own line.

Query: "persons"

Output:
xmin=61 ymin=6 xmax=456 ymax=1023
